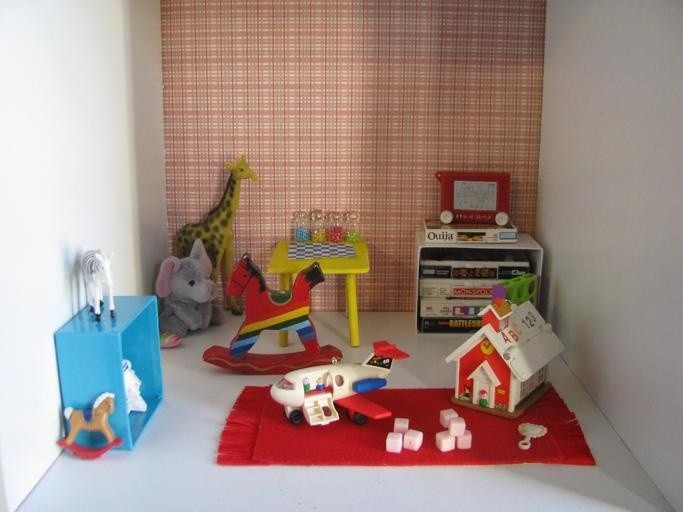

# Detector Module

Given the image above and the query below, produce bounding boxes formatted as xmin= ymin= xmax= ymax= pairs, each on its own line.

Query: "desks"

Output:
xmin=267 ymin=236 xmax=370 ymax=348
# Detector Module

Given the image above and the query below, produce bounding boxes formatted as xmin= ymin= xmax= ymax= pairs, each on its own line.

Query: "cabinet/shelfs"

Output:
xmin=53 ymin=295 xmax=165 ymax=451
xmin=412 ymin=234 xmax=544 ymax=337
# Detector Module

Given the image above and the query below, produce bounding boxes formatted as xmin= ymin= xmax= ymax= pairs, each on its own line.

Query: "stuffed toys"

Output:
xmin=159 ymin=330 xmax=182 ymax=349
xmin=121 ymin=358 xmax=148 ymax=414
xmin=150 ymin=237 xmax=226 ymax=336
xmin=173 ymin=155 xmax=257 ymax=316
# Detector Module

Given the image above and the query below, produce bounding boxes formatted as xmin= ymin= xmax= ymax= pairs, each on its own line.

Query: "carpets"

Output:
xmin=215 ymin=384 xmax=596 ymax=467
